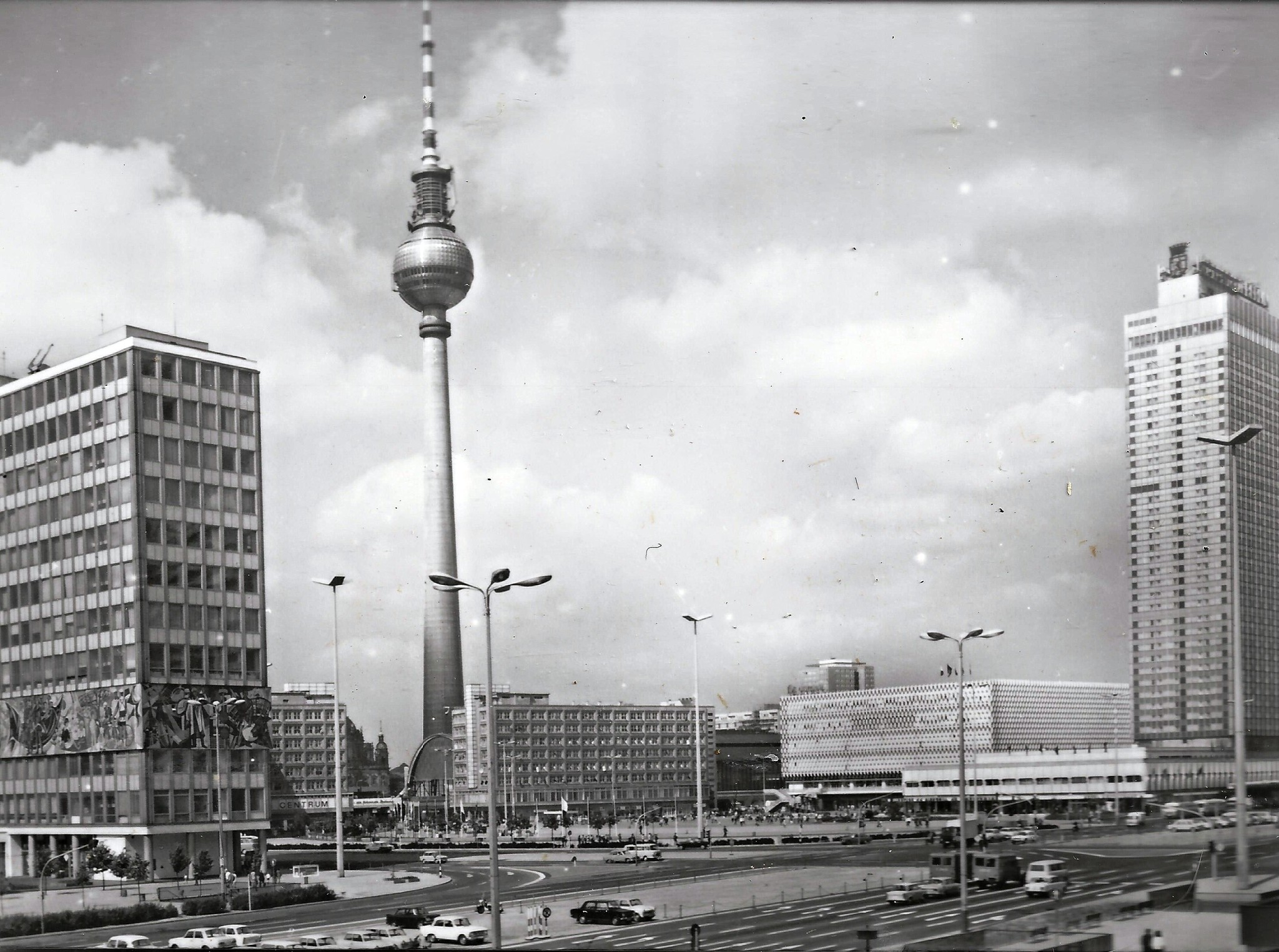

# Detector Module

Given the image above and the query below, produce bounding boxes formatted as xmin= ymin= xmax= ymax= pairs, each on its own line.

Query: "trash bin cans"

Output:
xmin=140 ymin=894 xmax=145 ymax=901
xmin=304 ymin=876 xmax=308 ymax=884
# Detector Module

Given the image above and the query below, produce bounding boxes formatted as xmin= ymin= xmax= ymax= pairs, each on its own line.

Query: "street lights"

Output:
xmin=683 ymin=613 xmax=716 ymax=838
xmin=428 ymin=566 xmax=554 ymax=952
xmin=604 ymin=754 xmax=623 ymax=839
xmin=962 ymin=685 xmax=994 ymax=818
xmin=188 ymin=696 xmax=247 ymax=900
xmin=921 ymin=627 xmax=1005 ymax=925
xmin=1101 ymin=693 xmax=1132 ymax=825
xmin=313 ymin=573 xmax=361 ymax=874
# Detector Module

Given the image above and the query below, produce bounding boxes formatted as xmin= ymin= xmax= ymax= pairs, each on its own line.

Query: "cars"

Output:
xmin=1126 ymin=796 xmax=1272 ymax=832
xmin=365 ymin=926 xmax=411 ymax=949
xmin=841 ymin=833 xmax=873 ymax=845
xmin=168 ymin=927 xmax=237 ymax=949
xmin=940 ymin=819 xmax=1038 ymax=847
xmin=295 ymin=934 xmax=350 ymax=950
xmin=886 ymin=850 xmax=1068 ymax=902
xmin=570 ymin=900 xmax=635 ymax=926
xmin=207 ymin=925 xmax=262 ymax=947
xmin=386 ymin=907 xmax=441 ymax=929
xmin=603 ymin=844 xmax=663 ymax=863
xmin=676 ymin=837 xmax=710 ymax=850
xmin=239 ymin=836 xmax=447 ymax=864
xmin=420 ymin=916 xmax=488 ymax=946
xmin=594 ymin=898 xmax=656 ymax=922
xmin=257 ymin=940 xmax=305 ymax=948
xmin=337 ymin=930 xmax=395 ymax=949
xmin=95 ymin=934 xmax=158 ymax=949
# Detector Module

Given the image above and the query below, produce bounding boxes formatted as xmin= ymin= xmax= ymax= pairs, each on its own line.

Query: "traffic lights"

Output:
xmin=706 ymin=830 xmax=710 ymax=842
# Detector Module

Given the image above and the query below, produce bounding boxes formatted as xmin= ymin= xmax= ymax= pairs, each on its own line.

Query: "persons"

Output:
xmin=192 ymin=868 xmax=199 ymax=886
xmin=576 ymin=816 xmax=678 ymax=845
xmin=905 ymin=816 xmax=930 ymax=827
xmin=225 ymin=867 xmax=282 ymax=891
xmin=680 ymin=804 xmax=823 ymax=829
xmin=1141 ymin=928 xmax=1165 ymax=952
